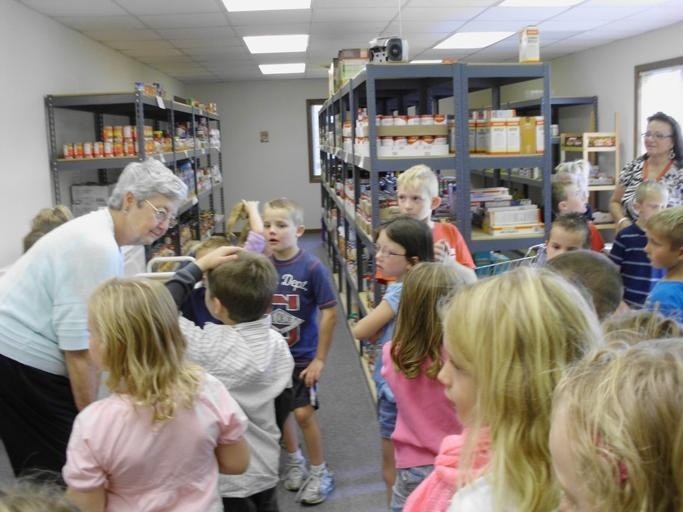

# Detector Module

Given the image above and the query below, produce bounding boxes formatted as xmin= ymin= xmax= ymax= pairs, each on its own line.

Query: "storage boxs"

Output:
xmin=323 ymin=47 xmax=369 ymax=97
xmin=69 ymin=180 xmax=116 ymax=219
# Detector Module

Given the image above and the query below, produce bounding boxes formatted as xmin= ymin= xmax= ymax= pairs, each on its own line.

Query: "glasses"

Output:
xmin=641 ymin=132 xmax=672 ymax=142
xmin=146 ymin=198 xmax=177 ymax=230
xmin=372 ymin=242 xmax=406 ymax=258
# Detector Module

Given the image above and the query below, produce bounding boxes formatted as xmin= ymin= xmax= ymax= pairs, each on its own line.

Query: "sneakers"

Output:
xmin=295 ymin=469 xmax=335 ymax=506
xmin=281 ymin=457 xmax=306 ymax=491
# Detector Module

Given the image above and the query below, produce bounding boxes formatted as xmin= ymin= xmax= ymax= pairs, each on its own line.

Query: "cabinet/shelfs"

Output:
xmin=44 ymin=89 xmax=226 ymax=268
xmin=318 ymin=60 xmax=550 ymax=412
xmin=558 ymin=109 xmax=624 ymax=242
xmin=460 ymin=93 xmax=601 ymax=221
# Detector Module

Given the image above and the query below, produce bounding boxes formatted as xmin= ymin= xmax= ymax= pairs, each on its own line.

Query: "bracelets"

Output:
xmin=617 ymin=217 xmax=630 ymax=228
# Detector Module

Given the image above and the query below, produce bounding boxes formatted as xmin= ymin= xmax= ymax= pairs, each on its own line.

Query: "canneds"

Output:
xmin=64 ymin=125 xmax=155 ymax=160
xmin=355 ymin=114 xmax=448 ymax=145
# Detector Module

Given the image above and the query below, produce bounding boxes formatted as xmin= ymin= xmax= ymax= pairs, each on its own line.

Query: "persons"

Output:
xmin=545 ymin=337 xmax=680 ymax=511
xmin=153 ymin=252 xmax=181 ymax=272
xmin=382 ymin=261 xmax=478 ymax=511
xmin=401 ymin=267 xmax=601 ymax=512
xmin=1 ymin=157 xmax=339 ymax=512
xmin=354 ymin=217 xmax=434 ymax=507
xmin=396 ymin=164 xmax=476 ymax=268
xmin=538 ymin=114 xmax=681 ymax=335
